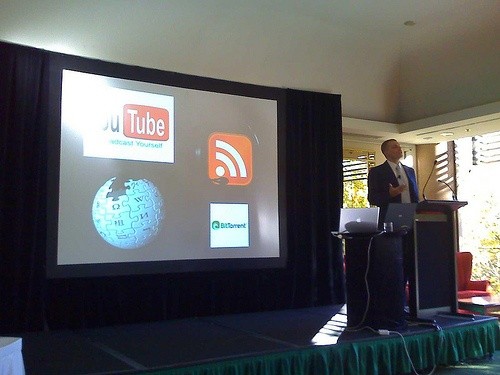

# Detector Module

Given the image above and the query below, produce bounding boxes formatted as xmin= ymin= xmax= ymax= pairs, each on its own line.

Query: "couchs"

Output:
xmin=457 ymin=252 xmax=492 ymax=314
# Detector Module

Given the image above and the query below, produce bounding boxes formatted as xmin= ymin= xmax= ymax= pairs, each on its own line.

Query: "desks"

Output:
xmin=457 ymin=296 xmax=500 ymax=317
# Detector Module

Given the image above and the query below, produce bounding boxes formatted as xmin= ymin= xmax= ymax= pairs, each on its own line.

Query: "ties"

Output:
xmin=396 ymin=163 xmax=409 ymax=203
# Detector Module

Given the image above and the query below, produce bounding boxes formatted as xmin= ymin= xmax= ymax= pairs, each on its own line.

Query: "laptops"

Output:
xmin=331 ymin=207 xmax=379 ymax=234
xmin=384 ymin=203 xmax=417 ymax=233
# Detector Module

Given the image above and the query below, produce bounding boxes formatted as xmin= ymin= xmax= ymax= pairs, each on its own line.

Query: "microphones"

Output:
xmin=438 ymin=180 xmax=457 ymax=200
xmin=398 ymin=175 xmax=401 ymax=178
xmin=423 ymin=160 xmax=437 ymax=200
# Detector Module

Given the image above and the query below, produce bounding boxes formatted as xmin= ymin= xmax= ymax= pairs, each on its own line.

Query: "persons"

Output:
xmin=367 ymin=139 xmax=420 ymax=315
